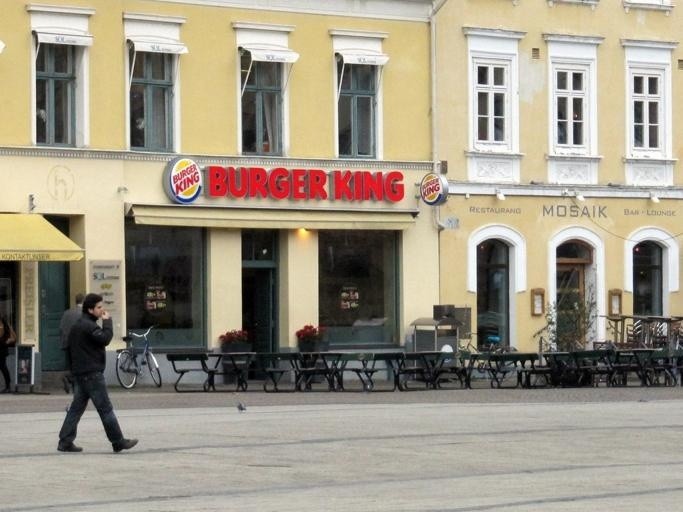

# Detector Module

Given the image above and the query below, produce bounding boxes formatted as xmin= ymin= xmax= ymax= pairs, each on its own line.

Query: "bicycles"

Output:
xmin=459 ymin=331 xmax=519 ymax=382
xmin=114 ymin=322 xmax=162 ymax=389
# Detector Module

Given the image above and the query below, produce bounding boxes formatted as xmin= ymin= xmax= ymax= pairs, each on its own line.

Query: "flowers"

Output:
xmin=292 ymin=323 xmax=328 ymax=339
xmin=214 ymin=329 xmax=249 ymax=345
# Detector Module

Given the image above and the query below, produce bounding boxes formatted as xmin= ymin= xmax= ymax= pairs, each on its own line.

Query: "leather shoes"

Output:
xmin=113 ymin=439 xmax=138 ymax=452
xmin=57 ymin=442 xmax=83 ymax=452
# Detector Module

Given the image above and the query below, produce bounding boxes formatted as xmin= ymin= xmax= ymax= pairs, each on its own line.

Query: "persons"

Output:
xmin=58 ymin=292 xmax=84 ymax=394
xmin=0 ymin=316 xmax=14 ymax=394
xmin=57 ymin=293 xmax=138 ymax=453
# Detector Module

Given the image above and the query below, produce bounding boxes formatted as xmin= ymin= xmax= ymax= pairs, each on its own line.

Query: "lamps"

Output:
xmin=493 ymin=186 xmax=507 ymax=201
xmin=647 ymin=192 xmax=660 ymax=203
xmin=572 ymin=188 xmax=585 ymax=203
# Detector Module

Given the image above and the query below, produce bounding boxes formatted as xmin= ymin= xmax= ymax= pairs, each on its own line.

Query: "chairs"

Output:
xmin=166 ymin=349 xmax=681 ymax=393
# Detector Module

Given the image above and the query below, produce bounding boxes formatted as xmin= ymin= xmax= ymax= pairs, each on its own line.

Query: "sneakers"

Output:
xmin=62 ymin=376 xmax=70 ymax=394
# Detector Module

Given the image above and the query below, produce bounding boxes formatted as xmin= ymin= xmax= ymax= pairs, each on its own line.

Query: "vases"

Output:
xmin=297 ymin=336 xmax=317 ymax=354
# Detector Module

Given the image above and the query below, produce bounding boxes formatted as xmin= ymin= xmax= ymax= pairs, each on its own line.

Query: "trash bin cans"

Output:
xmin=410 ymin=318 xmax=460 ymax=382
